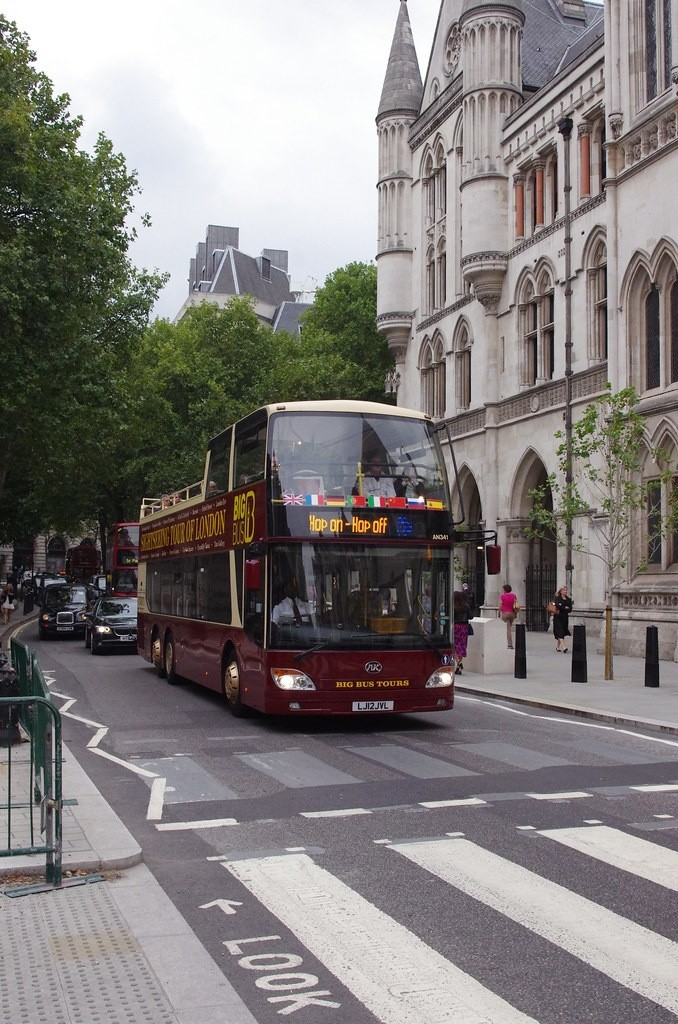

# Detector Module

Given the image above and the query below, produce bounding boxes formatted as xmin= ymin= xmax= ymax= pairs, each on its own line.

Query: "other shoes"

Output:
xmin=563 ymin=648 xmax=568 ymax=653
xmin=507 ymin=645 xmax=513 ymax=649
xmin=455 ymin=668 xmax=462 ymax=674
xmin=459 ymin=662 xmax=463 ymax=669
xmin=556 ymin=648 xmax=562 ymax=652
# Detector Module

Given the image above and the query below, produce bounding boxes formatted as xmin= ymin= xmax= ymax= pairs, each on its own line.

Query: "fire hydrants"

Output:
xmin=0 ymin=641 xmax=20 ymax=746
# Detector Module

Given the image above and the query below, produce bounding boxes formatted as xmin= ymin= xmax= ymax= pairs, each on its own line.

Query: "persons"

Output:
xmin=552 ymin=586 xmax=573 ymax=654
xmin=307 ymin=583 xmax=442 ymax=634
xmin=0 ymin=583 xmax=16 ymax=625
xmin=270 ymin=580 xmax=319 ymax=632
xmin=497 ymin=584 xmax=521 ymax=650
xmin=461 ymin=582 xmax=474 ymax=619
xmin=453 ymin=590 xmax=474 ymax=675
xmin=392 ymin=462 xmax=427 ymax=498
xmin=151 ymin=455 xmax=281 ymax=518
xmin=356 ymin=454 xmax=396 ymax=497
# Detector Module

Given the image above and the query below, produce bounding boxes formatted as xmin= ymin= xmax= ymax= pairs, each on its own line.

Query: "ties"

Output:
xmin=292 ymin=600 xmax=303 ymax=621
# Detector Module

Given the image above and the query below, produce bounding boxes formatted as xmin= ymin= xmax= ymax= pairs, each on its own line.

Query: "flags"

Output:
xmin=326 ymin=495 xmax=346 ymax=507
xmin=408 ymin=497 xmax=425 ymax=509
xmin=347 ymin=494 xmax=367 ymax=507
xmin=282 ymin=494 xmax=304 ymax=506
xmin=426 ymin=498 xmax=444 ymax=510
xmin=367 ymin=495 xmax=386 ymax=507
xmin=388 ymin=496 xmax=407 ymax=508
xmin=305 ymin=494 xmax=324 ymax=506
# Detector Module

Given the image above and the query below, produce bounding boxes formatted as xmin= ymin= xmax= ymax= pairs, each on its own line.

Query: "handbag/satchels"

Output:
xmin=468 ymin=619 xmax=474 ymax=635
xmin=513 ymin=596 xmax=520 ymax=613
xmin=545 ymin=596 xmax=561 ymax=615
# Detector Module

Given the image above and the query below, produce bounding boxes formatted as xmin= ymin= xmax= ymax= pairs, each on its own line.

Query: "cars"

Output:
xmin=84 ymin=596 xmax=139 ymax=655
xmin=13 ymin=567 xmax=65 ymax=607
xmin=85 ymin=575 xmax=107 ymax=599
xmin=38 ymin=583 xmax=88 ymax=637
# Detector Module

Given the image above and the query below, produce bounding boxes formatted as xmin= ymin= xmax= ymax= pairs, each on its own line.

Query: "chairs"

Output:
xmin=154 ymin=569 xmax=402 ymax=638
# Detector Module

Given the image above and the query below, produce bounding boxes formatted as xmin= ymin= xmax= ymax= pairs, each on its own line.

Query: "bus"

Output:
xmin=135 ymin=399 xmax=503 ymax=725
xmin=65 ymin=545 xmax=102 ymax=585
xmin=105 ymin=522 xmax=140 ymax=598
